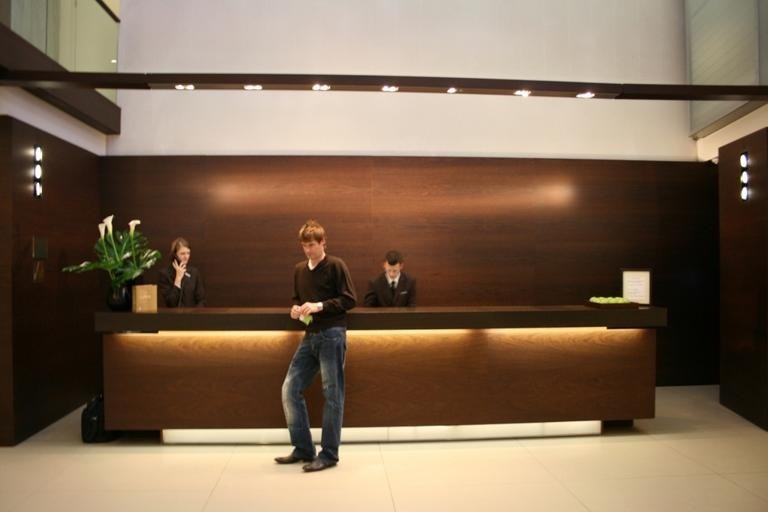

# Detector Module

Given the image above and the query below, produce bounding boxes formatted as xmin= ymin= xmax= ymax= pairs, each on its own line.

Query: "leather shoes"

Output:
xmin=303 ymin=455 xmax=337 ymax=471
xmin=274 ymin=445 xmax=316 ymax=462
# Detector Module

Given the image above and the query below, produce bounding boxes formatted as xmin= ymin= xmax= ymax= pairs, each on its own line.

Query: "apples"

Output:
xmin=589 ymin=296 xmax=634 ymax=304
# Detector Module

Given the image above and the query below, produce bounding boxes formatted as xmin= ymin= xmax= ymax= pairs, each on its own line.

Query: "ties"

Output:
xmin=391 ymin=281 xmax=397 ymax=297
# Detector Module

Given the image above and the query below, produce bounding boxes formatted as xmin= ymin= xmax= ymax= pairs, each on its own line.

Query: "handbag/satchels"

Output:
xmin=81 ymin=390 xmax=122 ymax=442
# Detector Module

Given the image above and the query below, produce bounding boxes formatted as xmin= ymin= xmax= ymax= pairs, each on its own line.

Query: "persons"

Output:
xmin=273 ymin=220 xmax=358 ymax=471
xmin=158 ymin=236 xmax=208 ymax=308
xmin=363 ymin=250 xmax=416 ymax=306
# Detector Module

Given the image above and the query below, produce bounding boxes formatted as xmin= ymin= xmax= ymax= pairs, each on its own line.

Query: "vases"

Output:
xmin=106 ymin=282 xmax=132 ymax=312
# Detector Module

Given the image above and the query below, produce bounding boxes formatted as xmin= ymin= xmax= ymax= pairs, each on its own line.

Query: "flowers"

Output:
xmin=59 ymin=214 xmax=160 ymax=288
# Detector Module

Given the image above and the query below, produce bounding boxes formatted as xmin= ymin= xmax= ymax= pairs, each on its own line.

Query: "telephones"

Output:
xmin=172 ymin=258 xmax=191 ymax=278
